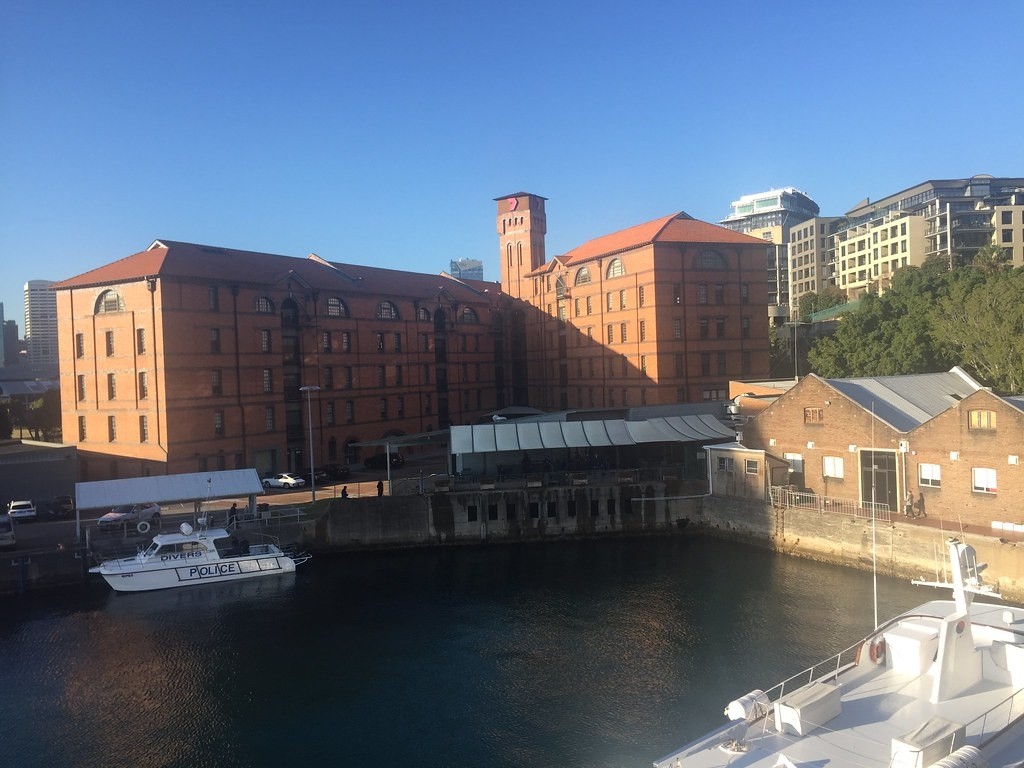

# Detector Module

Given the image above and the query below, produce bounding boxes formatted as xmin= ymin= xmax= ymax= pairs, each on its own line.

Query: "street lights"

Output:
xmin=299 ymin=386 xmax=321 ymax=503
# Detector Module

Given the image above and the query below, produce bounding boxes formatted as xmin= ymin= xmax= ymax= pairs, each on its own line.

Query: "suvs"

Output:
xmin=6 ymin=500 xmax=38 ymax=524
xmin=0 ymin=515 xmax=16 ymax=551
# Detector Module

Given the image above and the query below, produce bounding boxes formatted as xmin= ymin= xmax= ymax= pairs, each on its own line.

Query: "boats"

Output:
xmin=653 ymin=401 xmax=1023 ymax=768
xmin=86 ymin=478 xmax=313 ymax=593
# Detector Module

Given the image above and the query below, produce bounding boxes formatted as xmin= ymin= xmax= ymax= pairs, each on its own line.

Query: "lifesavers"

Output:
xmin=137 ymin=521 xmax=150 ymax=533
xmin=870 ymin=635 xmax=885 ymax=664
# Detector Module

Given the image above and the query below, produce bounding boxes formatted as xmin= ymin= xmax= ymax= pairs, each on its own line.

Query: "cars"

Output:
xmin=95 ymin=502 xmax=161 ymax=533
xmin=363 ymin=452 xmax=404 ymax=471
xmin=47 ymin=495 xmax=74 ymax=522
xmin=295 ymin=467 xmax=328 ymax=486
xmin=320 ymin=463 xmax=353 ymax=482
xmin=261 ymin=473 xmax=306 ymax=490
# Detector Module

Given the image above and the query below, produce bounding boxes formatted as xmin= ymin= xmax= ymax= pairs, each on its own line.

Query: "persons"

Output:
xmin=377 ymin=479 xmax=383 ymax=496
xmin=342 ymin=486 xmax=348 ymax=498
xmin=905 ymin=491 xmax=915 ymax=519
xmin=228 ymin=503 xmax=241 ymax=529
xmin=58 ymin=542 xmax=64 ymax=549
xmin=225 ymin=537 xmax=250 ymax=557
